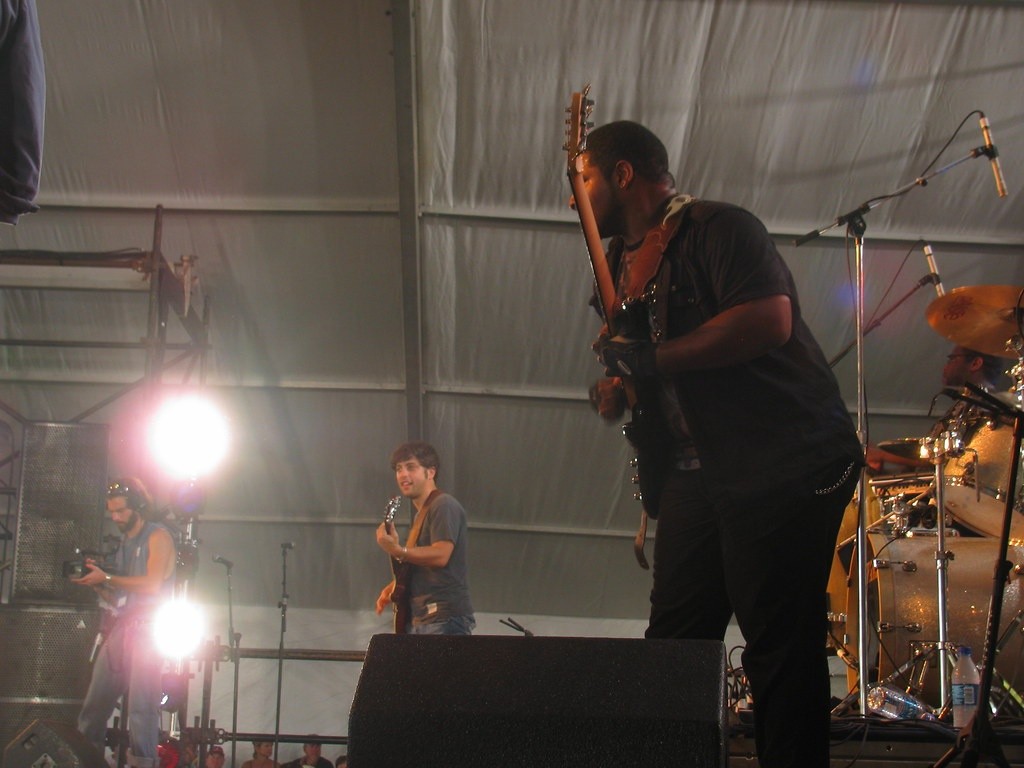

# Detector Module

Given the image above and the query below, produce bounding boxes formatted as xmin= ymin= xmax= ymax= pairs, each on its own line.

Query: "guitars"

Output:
xmin=62 ymin=560 xmax=122 ymax=612
xmin=554 ymin=73 xmax=667 ymax=520
xmin=381 ymin=493 xmax=412 ymax=634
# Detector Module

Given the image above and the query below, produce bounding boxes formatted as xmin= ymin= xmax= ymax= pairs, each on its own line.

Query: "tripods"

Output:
xmin=830 ymin=450 xmax=1024 ymax=768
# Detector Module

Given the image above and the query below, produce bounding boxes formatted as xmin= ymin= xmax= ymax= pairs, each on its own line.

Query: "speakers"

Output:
xmin=346 ymin=633 xmax=730 ymax=768
xmin=0 ymin=717 xmax=111 ymax=768
xmin=0 ymin=601 xmax=107 ymax=753
xmin=10 ymin=421 xmax=110 ymax=608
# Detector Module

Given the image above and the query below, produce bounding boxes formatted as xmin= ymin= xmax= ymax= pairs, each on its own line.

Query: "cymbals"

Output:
xmin=877 ymin=437 xmax=938 ymax=460
xmin=925 ymin=283 xmax=1024 ymax=360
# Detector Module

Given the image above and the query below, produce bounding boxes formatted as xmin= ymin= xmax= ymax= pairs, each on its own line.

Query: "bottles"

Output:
xmin=867 ymin=687 xmax=936 ymax=720
xmin=951 ymin=646 xmax=979 ymax=729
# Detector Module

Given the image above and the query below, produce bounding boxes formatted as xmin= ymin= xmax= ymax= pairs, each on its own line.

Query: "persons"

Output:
xmin=106 ymin=733 xmax=348 ymax=768
xmin=568 ymin=118 xmax=867 ymax=768
xmin=375 ymin=441 xmax=477 ymax=637
xmin=69 ymin=476 xmax=177 ymax=768
xmin=928 ymin=345 xmax=1009 ymax=443
xmin=0 ymin=0 xmax=47 ymax=224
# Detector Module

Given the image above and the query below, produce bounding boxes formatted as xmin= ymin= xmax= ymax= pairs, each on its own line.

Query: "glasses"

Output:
xmin=107 ymin=483 xmax=129 ymax=496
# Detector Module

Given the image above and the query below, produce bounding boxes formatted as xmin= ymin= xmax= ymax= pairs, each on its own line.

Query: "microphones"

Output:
xmin=282 ymin=541 xmax=296 ymax=549
xmin=923 ymin=240 xmax=945 ymax=298
xmin=213 ymin=555 xmax=235 ymax=569
xmin=979 ymin=112 xmax=1009 ymax=198
xmin=856 ymin=667 xmax=880 ymax=688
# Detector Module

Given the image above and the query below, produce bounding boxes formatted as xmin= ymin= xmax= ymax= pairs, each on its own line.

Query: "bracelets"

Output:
xmin=396 ymin=548 xmax=407 ymax=564
xmin=106 ymin=573 xmax=110 ymax=586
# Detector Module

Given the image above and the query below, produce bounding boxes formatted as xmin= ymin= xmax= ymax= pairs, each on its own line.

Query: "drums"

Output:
xmin=825 ymin=469 xmax=888 ymax=674
xmin=843 ymin=527 xmax=1024 ymax=708
xmin=928 ymin=411 xmax=1024 ymax=545
